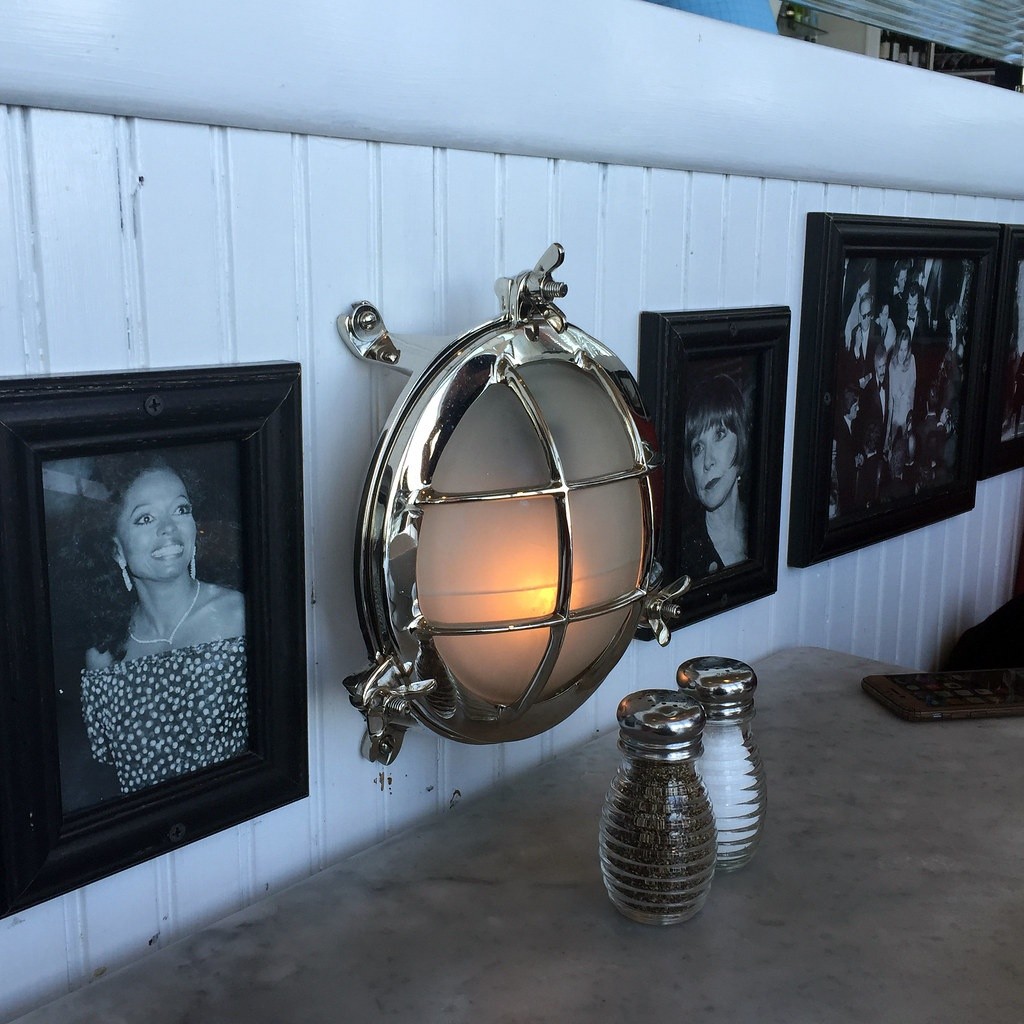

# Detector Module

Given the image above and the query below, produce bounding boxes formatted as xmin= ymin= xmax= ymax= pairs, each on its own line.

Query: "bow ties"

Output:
xmin=908 ymin=316 xmax=915 ymax=322
xmin=879 ymin=380 xmax=886 ymax=392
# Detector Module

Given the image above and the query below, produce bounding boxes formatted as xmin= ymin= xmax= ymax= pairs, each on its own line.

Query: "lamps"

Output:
xmin=335 ymin=244 xmax=691 ymax=765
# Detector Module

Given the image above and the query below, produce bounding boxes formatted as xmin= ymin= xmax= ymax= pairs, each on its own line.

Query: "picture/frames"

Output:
xmin=787 ymin=213 xmax=1002 ymax=569
xmin=977 ymin=224 xmax=1024 ymax=480
xmin=0 ymin=360 xmax=309 ymax=922
xmin=636 ymin=306 xmax=793 ymax=641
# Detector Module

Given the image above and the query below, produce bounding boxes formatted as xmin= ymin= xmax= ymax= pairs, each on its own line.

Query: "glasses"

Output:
xmin=861 ymin=310 xmax=872 ymax=320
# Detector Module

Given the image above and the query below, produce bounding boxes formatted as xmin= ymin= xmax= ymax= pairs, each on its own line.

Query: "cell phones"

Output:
xmin=861 ymin=667 xmax=1024 ymax=723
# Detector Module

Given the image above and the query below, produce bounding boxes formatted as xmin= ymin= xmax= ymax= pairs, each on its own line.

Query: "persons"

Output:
xmin=671 ymin=373 xmax=755 ymax=574
xmin=77 ymin=454 xmax=248 ymax=793
xmin=828 ymin=256 xmax=968 ymax=505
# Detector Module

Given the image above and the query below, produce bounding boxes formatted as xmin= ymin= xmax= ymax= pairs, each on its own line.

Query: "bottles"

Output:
xmin=677 ymin=656 xmax=766 ymax=871
xmin=599 ymin=689 xmax=718 ymax=925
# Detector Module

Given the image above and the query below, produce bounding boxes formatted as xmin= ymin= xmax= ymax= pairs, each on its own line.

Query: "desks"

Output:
xmin=0 ymin=647 xmax=1024 ymax=1024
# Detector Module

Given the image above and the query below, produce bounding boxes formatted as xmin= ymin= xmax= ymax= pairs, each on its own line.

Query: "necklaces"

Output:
xmin=128 ymin=578 xmax=201 ymax=649
